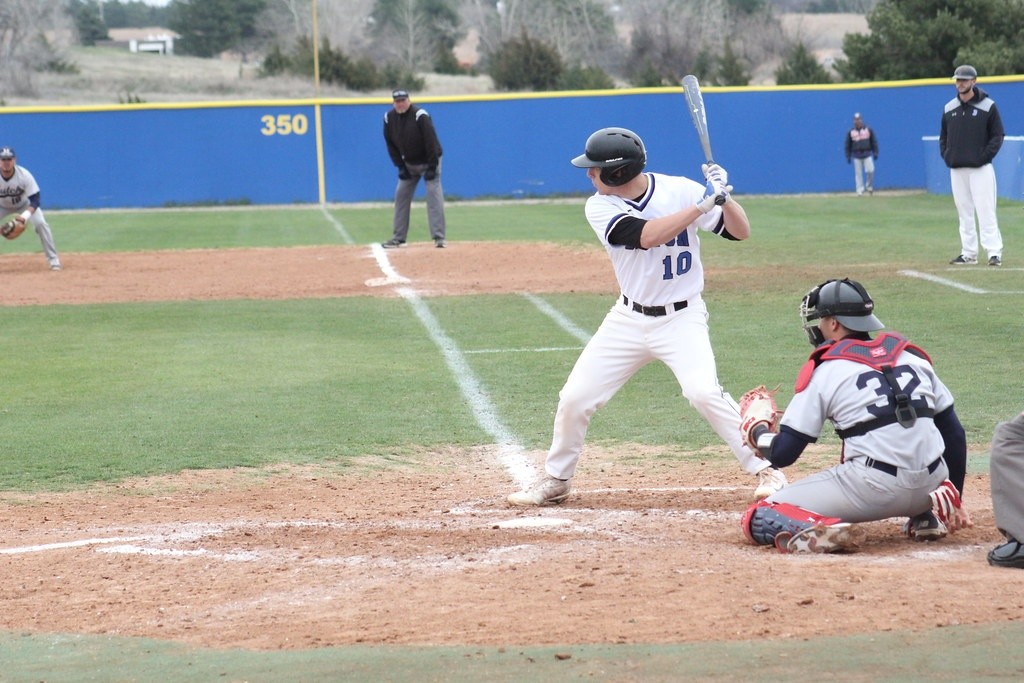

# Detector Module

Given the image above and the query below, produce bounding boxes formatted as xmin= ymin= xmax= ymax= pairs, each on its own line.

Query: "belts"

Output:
xmin=623 ymin=295 xmax=688 ymax=316
xmin=866 ymin=454 xmax=943 ymax=476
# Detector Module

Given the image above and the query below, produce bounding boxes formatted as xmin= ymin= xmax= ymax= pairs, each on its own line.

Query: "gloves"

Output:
xmin=701 ymin=163 xmax=728 ymax=185
xmin=424 ymin=167 xmax=435 ymax=180
xmin=398 ymin=166 xmax=410 ymax=179
xmin=695 ymin=181 xmax=733 ymax=213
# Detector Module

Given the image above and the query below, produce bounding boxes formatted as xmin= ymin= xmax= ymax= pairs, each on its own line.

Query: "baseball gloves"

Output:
xmin=0 ymin=215 xmax=27 ymax=240
xmin=738 ymin=384 xmax=779 ymax=461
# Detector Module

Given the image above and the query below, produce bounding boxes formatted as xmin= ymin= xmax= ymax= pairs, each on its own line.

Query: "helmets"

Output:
xmin=571 ymin=127 xmax=647 ymax=187
xmin=815 ymin=279 xmax=885 ymax=332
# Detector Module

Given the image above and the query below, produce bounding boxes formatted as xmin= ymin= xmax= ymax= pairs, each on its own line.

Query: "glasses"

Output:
xmin=2 ymin=158 xmax=11 ymax=161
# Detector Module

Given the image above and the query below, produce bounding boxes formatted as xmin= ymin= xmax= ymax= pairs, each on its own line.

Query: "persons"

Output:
xmin=939 ymin=65 xmax=1005 ymax=266
xmin=845 ymin=112 xmax=878 ymax=197
xmin=985 ymin=411 xmax=1024 ymax=568
xmin=0 ymin=147 xmax=61 ymax=271
xmin=738 ymin=280 xmax=966 ymax=554
xmin=382 ymin=89 xmax=448 ymax=247
xmin=507 ymin=127 xmax=787 ymax=507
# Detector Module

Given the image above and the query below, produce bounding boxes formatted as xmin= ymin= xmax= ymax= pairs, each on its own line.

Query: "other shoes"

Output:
xmin=382 ymin=238 xmax=407 ymax=248
xmin=433 ymin=237 xmax=448 ymax=247
xmin=47 ymin=257 xmax=61 ymax=271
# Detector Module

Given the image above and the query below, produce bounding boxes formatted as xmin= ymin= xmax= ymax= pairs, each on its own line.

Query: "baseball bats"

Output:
xmin=681 ymin=73 xmax=725 ymax=206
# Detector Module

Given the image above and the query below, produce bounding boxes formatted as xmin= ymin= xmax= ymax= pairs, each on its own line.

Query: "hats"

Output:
xmin=951 ymin=65 xmax=977 ymax=81
xmin=0 ymin=147 xmax=15 ymax=158
xmin=391 ymin=89 xmax=408 ymax=99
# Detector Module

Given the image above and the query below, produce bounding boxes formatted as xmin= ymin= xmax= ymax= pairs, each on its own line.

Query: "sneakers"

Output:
xmin=986 ymin=256 xmax=1001 ymax=266
xmin=507 ymin=474 xmax=571 ymax=509
xmin=906 ymin=514 xmax=948 ymax=541
xmin=948 ymin=254 xmax=978 ymax=265
xmin=752 ymin=467 xmax=788 ymax=503
xmin=786 ymin=520 xmax=867 ymax=556
